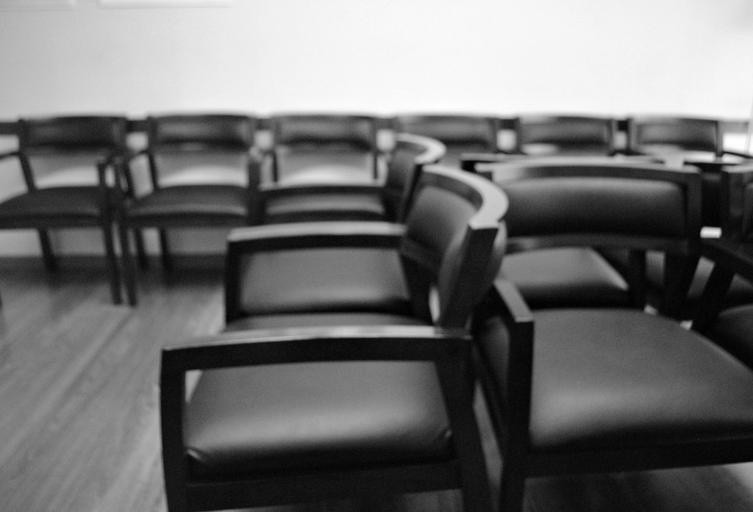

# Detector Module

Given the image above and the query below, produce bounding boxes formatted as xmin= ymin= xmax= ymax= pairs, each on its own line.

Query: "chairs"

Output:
xmin=0 ymin=109 xmax=753 ymax=512
